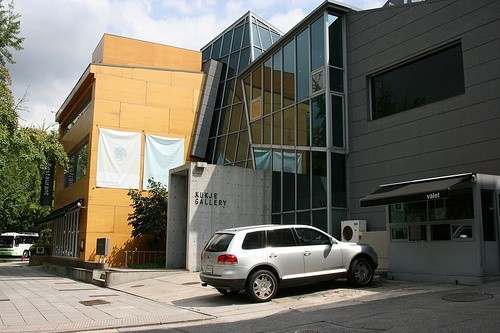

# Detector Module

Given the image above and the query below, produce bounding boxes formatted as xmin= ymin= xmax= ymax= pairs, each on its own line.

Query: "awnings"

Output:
xmin=34 ymin=199 xmax=80 ymax=224
xmin=359 ymin=172 xmax=473 ymax=208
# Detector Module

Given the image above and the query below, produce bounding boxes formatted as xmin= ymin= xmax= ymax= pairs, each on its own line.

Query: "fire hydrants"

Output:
xmin=21 ymin=256 xmax=24 ymax=261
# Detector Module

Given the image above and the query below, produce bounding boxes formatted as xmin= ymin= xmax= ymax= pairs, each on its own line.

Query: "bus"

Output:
xmin=0 ymin=232 xmax=39 ymax=258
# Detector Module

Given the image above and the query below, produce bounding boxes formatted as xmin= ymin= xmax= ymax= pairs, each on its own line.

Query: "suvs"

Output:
xmin=199 ymin=224 xmax=378 ymax=301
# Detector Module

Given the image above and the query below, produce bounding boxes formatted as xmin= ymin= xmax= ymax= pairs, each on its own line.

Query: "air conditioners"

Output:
xmin=341 ymin=219 xmax=366 ymax=242
